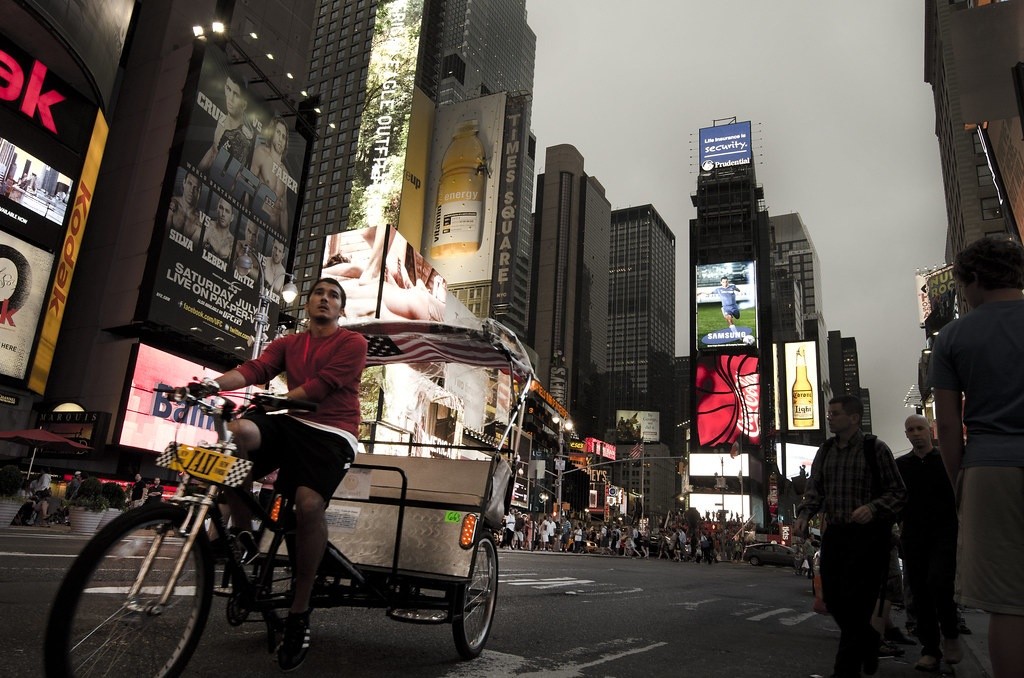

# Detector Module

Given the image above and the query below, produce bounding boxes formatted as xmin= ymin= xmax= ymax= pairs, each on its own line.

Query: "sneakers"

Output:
xmin=879 ymin=641 xmax=904 ymax=658
xmin=276 ymin=609 xmax=310 ymax=672
xmin=225 ymin=526 xmax=245 ymax=556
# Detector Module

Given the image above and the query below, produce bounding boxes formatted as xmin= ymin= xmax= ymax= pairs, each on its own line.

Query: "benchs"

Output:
xmin=328 ymin=439 xmax=518 ymax=526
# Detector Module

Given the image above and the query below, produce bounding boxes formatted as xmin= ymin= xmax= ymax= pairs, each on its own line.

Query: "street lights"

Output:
xmin=552 ymin=412 xmax=574 ymax=523
xmin=677 ymin=420 xmax=691 ymax=476
xmin=235 ymin=243 xmax=298 ymax=406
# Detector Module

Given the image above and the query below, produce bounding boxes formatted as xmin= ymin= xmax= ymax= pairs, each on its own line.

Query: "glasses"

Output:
xmin=827 ymin=412 xmax=847 ymax=418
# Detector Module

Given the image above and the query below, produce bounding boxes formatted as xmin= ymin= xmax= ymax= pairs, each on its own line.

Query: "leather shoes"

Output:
xmin=946 ymin=640 xmax=964 ymax=664
xmin=915 ymin=655 xmax=940 ymax=672
xmin=884 ymin=625 xmax=917 ymax=645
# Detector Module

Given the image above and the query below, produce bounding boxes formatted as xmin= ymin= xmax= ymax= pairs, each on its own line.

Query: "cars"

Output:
xmin=743 ymin=543 xmax=794 ymax=566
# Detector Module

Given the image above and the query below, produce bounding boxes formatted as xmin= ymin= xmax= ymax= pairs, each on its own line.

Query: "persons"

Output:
xmin=188 ymin=278 xmax=369 ymax=677
xmin=34 ymin=466 xmax=52 ymax=493
xmin=253 ymin=114 xmax=290 ymax=238
xmin=697 ymin=276 xmax=740 ymax=338
xmin=322 ymin=253 xmax=364 ymax=280
xmin=382 ymin=276 xmax=445 ymax=322
xmin=808 ymin=390 xmax=905 ymax=676
xmin=358 ymin=226 xmax=397 ymax=286
xmin=147 ymin=478 xmax=165 ymax=502
xmin=71 ymin=470 xmax=82 ymax=495
xmin=205 ymin=195 xmax=234 ymax=261
xmin=129 ymin=474 xmax=146 ymax=508
xmin=35 ymin=489 xmax=52 ymax=528
xmin=259 ymin=236 xmax=287 ymax=292
xmin=928 ymin=236 xmax=1024 ymax=677
xmin=500 ymin=493 xmax=827 ymax=580
xmin=164 ymin=170 xmax=200 ymax=245
xmin=863 ymin=531 xmax=917 ymax=660
xmin=233 ymin=217 xmax=260 ymax=284
xmin=196 ymin=74 xmax=253 ymax=203
xmin=885 ymin=412 xmax=965 ymax=670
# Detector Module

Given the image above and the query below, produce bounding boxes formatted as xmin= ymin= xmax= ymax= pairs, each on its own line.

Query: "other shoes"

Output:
xmin=864 ymin=634 xmax=882 ymax=675
xmin=958 ymin=618 xmax=970 ymax=634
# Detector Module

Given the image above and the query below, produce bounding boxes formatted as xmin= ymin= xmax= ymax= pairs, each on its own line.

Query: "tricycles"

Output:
xmin=42 ymin=321 xmax=537 ymax=678
